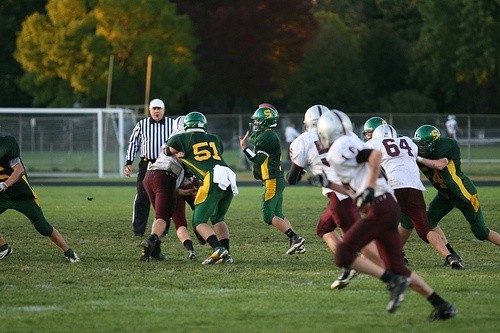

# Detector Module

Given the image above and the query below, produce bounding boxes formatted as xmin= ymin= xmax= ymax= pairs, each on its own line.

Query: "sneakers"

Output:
xmin=187 ymin=252 xmax=196 ymax=260
xmin=446 ymin=254 xmax=465 ymax=269
xmin=286 ymin=236 xmax=307 ymax=255
xmin=141 ymin=235 xmax=164 ymax=262
xmin=215 ymin=255 xmax=234 ymax=264
xmin=428 ymin=303 xmax=457 ymax=320
xmin=201 ymin=248 xmax=228 ymax=264
xmin=402 ymin=248 xmax=409 ymax=264
xmin=331 ymin=268 xmax=358 ymax=290
xmin=0 ymin=247 xmax=13 ymax=259
xmin=386 ymin=276 xmax=412 ymax=314
xmin=63 ymin=249 xmax=79 ymax=263
xmin=443 ymin=252 xmax=462 ymax=266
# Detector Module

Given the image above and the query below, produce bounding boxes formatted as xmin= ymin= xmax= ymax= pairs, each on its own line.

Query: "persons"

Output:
xmin=240 ymin=103 xmax=307 ymax=254
xmin=284 ymin=104 xmax=464 ymax=321
xmin=0 ymin=136 xmax=79 ymax=264
xmin=139 ymin=111 xmax=238 ymax=264
xmin=125 ymin=98 xmax=178 ymax=237
xmin=413 ymin=124 xmax=500 ymax=262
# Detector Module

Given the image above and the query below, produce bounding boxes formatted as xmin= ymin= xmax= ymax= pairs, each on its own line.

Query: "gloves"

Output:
xmin=355 ymin=187 xmax=374 ymax=209
xmin=0 ymin=182 xmax=8 ymax=193
xmin=307 ymin=167 xmax=329 ymax=187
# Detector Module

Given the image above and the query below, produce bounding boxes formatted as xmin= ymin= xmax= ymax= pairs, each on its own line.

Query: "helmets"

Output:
xmin=372 ymin=125 xmax=397 ymax=139
xmin=316 ymin=109 xmax=353 ymax=148
xmin=184 ymin=111 xmax=207 ymax=132
xmin=174 ymin=116 xmax=186 ymax=134
xmin=362 ymin=117 xmax=386 ymax=144
xmin=303 ymin=105 xmax=331 ymax=131
xmin=252 ymin=106 xmax=280 ymax=131
xmin=411 ymin=124 xmax=441 ymax=153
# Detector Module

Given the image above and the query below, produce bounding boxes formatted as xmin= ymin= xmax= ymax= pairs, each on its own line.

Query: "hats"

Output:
xmin=149 ymin=99 xmax=164 ymax=108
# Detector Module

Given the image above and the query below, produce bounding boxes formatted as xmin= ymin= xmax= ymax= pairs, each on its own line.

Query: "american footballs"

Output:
xmin=179 ymin=176 xmax=203 ymax=190
